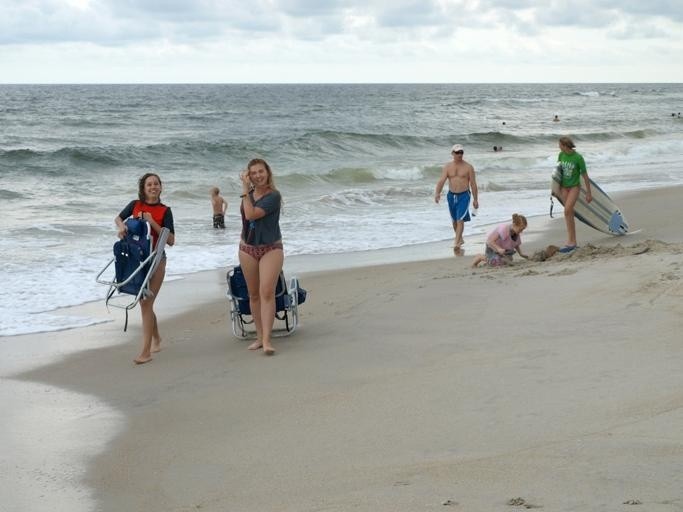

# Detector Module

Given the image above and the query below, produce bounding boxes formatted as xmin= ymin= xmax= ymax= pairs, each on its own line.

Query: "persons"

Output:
xmin=238 ymin=158 xmax=283 ymax=354
xmin=494 ymin=145 xmax=499 ymax=152
xmin=114 ymin=171 xmax=175 ymax=364
xmin=472 ymin=214 xmax=529 ymax=268
xmin=434 ymin=144 xmax=479 ymax=254
xmin=557 ymin=137 xmax=592 ymax=254
xmin=210 ymin=186 xmax=227 ymax=228
xmin=554 ymin=115 xmax=560 ymax=122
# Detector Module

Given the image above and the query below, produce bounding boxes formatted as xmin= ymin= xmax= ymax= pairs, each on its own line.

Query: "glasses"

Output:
xmin=455 ymin=151 xmax=463 ymax=154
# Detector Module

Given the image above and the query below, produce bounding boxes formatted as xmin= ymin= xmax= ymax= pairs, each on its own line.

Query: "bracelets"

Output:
xmin=240 ymin=193 xmax=249 ymax=198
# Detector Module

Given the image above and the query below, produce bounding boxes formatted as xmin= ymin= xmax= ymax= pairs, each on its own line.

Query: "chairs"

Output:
xmin=225 ymin=268 xmax=307 ymax=340
xmin=96 ymin=218 xmax=173 ymax=310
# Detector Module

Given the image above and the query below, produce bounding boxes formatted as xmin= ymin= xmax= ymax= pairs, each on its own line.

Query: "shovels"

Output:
xmin=495 ymin=249 xmax=516 ymax=255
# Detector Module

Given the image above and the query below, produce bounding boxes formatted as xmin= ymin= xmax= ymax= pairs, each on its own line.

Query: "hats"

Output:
xmin=451 ymin=144 xmax=464 ymax=153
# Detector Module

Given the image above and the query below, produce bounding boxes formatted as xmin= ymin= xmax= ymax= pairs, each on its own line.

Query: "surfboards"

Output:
xmin=551 ymin=161 xmax=629 ymax=236
